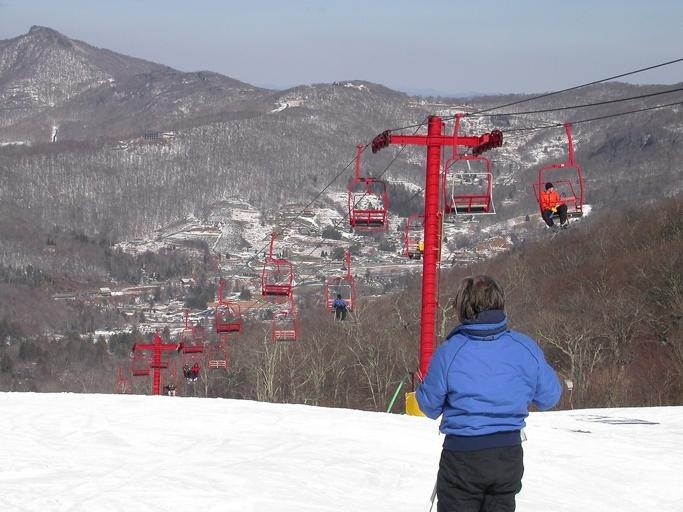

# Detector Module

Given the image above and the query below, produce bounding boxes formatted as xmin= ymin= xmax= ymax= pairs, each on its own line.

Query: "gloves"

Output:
xmin=551 ymin=207 xmax=558 ymax=214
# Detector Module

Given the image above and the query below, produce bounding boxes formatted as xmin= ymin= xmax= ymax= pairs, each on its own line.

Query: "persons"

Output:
xmin=188 ymin=362 xmax=201 ymax=382
xmin=416 ymin=274 xmax=564 ymax=512
xmin=418 ymin=238 xmax=424 ymax=252
xmin=184 ymin=363 xmax=192 ymax=385
xmin=170 ymin=384 xmax=176 ymax=396
xmin=540 ymin=181 xmax=572 ymax=232
xmin=333 ymin=294 xmax=348 ymax=321
xmin=167 ymin=384 xmax=170 ymax=396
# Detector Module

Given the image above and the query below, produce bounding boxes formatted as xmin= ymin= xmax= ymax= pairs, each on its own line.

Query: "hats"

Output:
xmin=544 ymin=182 xmax=554 ymax=190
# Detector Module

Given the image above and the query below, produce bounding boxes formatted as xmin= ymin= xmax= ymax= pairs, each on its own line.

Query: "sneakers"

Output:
xmin=552 ymin=219 xmax=569 ymax=233
